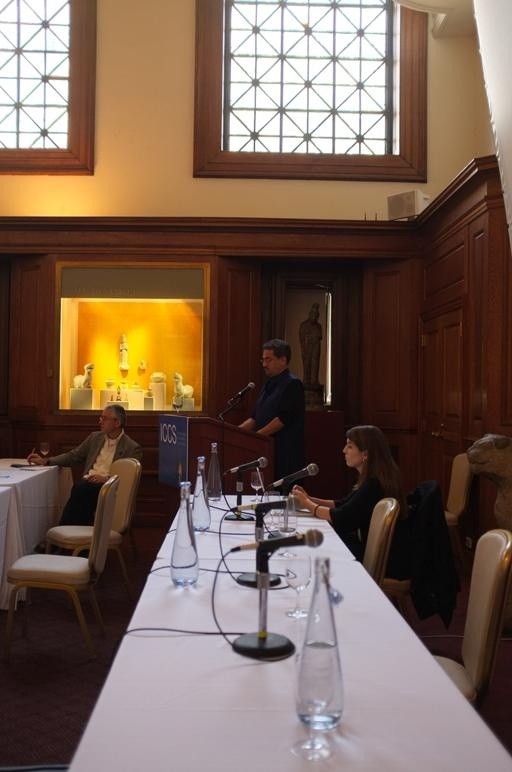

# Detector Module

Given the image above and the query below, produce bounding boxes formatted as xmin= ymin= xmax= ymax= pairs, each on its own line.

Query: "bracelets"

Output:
xmin=313 ymin=503 xmax=321 ymax=518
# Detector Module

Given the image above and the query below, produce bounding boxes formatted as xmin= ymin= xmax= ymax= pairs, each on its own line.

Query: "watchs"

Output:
xmin=47 ymin=457 xmax=52 ymax=466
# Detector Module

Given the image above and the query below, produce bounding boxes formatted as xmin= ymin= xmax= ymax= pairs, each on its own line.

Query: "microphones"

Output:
xmin=273 ymin=463 xmax=319 ymax=487
xmin=224 ymin=457 xmax=269 ymax=475
xmin=231 ymin=497 xmax=302 ymax=511
xmin=228 ymin=382 xmax=256 ymax=401
xmin=231 ymin=529 xmax=323 ymax=553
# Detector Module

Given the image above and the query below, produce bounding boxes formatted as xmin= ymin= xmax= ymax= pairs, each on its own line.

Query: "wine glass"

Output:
xmin=278 ymin=511 xmax=299 ymax=557
xmin=286 ymin=555 xmax=311 ymax=618
xmin=264 ymin=491 xmax=280 ymax=526
xmin=40 ymin=442 xmax=49 ymax=458
xmin=293 ymin=652 xmax=332 ymax=762
xmin=250 ymin=471 xmax=263 ymax=504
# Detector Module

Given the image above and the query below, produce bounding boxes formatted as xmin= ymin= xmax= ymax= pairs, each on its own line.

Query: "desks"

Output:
xmin=0 ymin=458 xmax=73 ymax=624
xmin=68 ymin=494 xmax=512 ymax=772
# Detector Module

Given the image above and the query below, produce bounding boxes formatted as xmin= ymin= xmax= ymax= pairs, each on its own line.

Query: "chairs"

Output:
xmin=442 ymin=452 xmax=471 ymax=577
xmin=46 ymin=458 xmax=141 ymax=606
xmin=362 ymin=498 xmax=399 ymax=587
xmin=6 ymin=476 xmax=121 ymax=640
xmin=382 ymin=480 xmax=436 ymax=627
xmin=430 ymin=529 xmax=512 ymax=709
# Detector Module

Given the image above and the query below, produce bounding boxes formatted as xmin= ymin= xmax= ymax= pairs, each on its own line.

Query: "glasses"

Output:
xmin=258 ymin=355 xmax=279 ymax=364
xmin=99 ymin=416 xmax=117 ymax=420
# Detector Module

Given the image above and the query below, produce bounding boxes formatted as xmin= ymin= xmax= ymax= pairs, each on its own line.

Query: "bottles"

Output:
xmin=171 ymin=481 xmax=199 ymax=587
xmin=206 ymin=442 xmax=224 ymax=500
xmin=295 ymin=558 xmax=345 ymax=731
xmin=190 ymin=455 xmax=212 ymax=532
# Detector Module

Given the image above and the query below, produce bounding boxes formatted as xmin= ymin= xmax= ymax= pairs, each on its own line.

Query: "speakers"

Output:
xmin=386 ymin=189 xmax=432 ymax=221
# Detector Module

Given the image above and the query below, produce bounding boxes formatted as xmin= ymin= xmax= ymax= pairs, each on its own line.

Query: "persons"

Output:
xmin=236 ymin=339 xmax=307 ymax=498
xmin=298 ymin=302 xmax=323 ymax=384
xmin=291 ymin=425 xmax=413 ymax=581
xmin=26 ymin=404 xmax=144 ymax=554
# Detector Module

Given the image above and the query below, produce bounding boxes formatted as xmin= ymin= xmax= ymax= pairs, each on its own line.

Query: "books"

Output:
xmin=270 ymin=494 xmax=316 ymax=516
